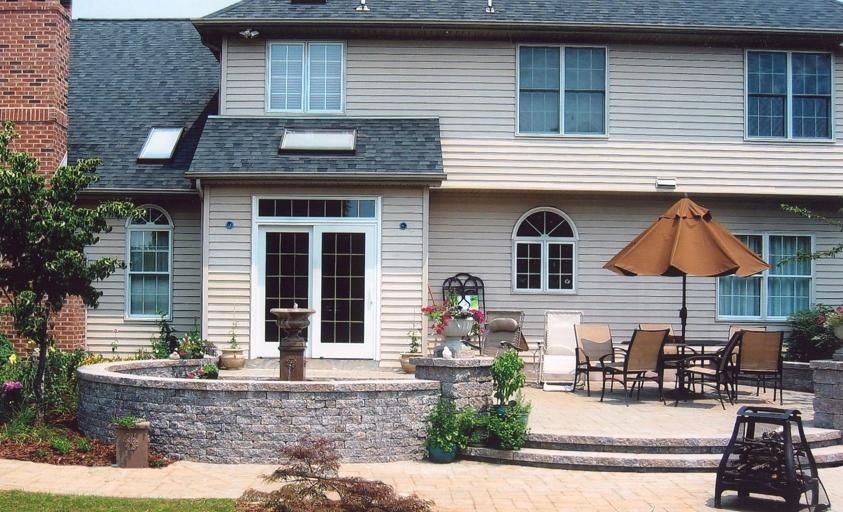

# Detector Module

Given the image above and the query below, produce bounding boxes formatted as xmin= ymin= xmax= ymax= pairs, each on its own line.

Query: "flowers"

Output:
xmin=421 ymin=292 xmax=490 ymax=338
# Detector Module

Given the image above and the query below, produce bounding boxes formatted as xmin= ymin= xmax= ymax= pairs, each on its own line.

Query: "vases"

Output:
xmin=440 ymin=317 xmax=474 ymax=358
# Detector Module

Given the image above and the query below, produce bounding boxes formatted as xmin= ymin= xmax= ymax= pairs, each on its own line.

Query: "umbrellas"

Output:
xmin=601 ymin=193 xmax=773 ymax=387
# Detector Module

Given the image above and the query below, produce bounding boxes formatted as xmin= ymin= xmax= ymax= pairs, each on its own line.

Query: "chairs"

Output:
xmin=538 ymin=310 xmax=784 ymax=409
xmin=482 ymin=310 xmax=529 ymax=357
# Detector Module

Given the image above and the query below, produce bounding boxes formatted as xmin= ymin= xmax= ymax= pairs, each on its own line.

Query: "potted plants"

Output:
xmin=221 ymin=303 xmax=247 ymax=370
xmin=421 ymin=349 xmax=534 ymax=463
xmin=400 ymin=302 xmax=423 ymax=374
